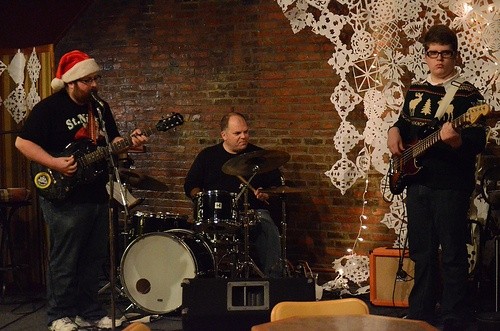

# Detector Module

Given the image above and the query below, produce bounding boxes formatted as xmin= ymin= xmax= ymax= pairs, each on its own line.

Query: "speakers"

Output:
xmin=181 ymin=278 xmax=316 ymax=331
xmin=368 ymin=247 xmax=416 ymax=307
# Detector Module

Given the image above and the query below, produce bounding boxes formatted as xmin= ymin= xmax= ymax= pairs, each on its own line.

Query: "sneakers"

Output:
xmin=75 ymin=314 xmax=122 ymax=328
xmin=48 ymin=317 xmax=89 ymax=331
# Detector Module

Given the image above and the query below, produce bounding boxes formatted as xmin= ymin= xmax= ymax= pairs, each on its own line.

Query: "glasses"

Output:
xmin=427 ymin=50 xmax=456 ymax=58
xmin=74 ymin=75 xmax=101 ymax=85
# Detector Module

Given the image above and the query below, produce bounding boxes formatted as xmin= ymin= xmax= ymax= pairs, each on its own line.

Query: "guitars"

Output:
xmin=28 ymin=110 xmax=184 ymax=200
xmin=387 ymin=98 xmax=492 ymax=195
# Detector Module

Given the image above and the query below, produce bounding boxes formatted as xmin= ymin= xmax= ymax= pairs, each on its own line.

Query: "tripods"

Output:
xmin=230 ymin=186 xmax=267 ymax=281
xmin=269 ymin=194 xmax=302 ymax=279
xmin=96 ymin=105 xmax=135 ymax=331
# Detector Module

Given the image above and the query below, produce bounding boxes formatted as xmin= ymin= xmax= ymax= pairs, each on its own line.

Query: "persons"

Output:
xmin=387 ymin=24 xmax=486 ymax=331
xmin=15 ymin=49 xmax=150 ymax=331
xmin=184 ymin=111 xmax=283 ymax=281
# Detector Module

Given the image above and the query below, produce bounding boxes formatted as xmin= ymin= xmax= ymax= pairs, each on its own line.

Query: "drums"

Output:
xmin=130 ymin=209 xmax=189 ymax=237
xmin=218 ymin=232 xmax=258 ymax=264
xmin=192 ymin=189 xmax=241 ymax=234
xmin=117 ymin=228 xmax=216 ymax=315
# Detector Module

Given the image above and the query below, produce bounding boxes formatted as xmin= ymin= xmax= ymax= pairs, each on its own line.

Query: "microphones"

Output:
xmin=398 ymin=270 xmax=407 ymax=278
xmin=90 ymin=91 xmax=105 ymax=108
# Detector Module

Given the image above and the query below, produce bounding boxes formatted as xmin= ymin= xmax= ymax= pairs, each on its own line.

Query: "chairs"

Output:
xmin=271 ymin=298 xmax=370 ymax=321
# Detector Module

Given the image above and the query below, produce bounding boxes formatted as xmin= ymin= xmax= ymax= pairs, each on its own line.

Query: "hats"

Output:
xmin=50 ymin=50 xmax=99 ymax=91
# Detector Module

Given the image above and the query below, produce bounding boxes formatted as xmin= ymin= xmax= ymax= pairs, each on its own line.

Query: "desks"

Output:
xmin=252 ymin=315 xmax=440 ymax=331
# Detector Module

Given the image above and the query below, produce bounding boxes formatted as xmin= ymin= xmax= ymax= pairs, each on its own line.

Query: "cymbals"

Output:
xmin=259 ymin=186 xmax=320 ymax=195
xmin=221 ymin=149 xmax=290 ymax=176
xmin=112 ymin=170 xmax=170 ymax=191
